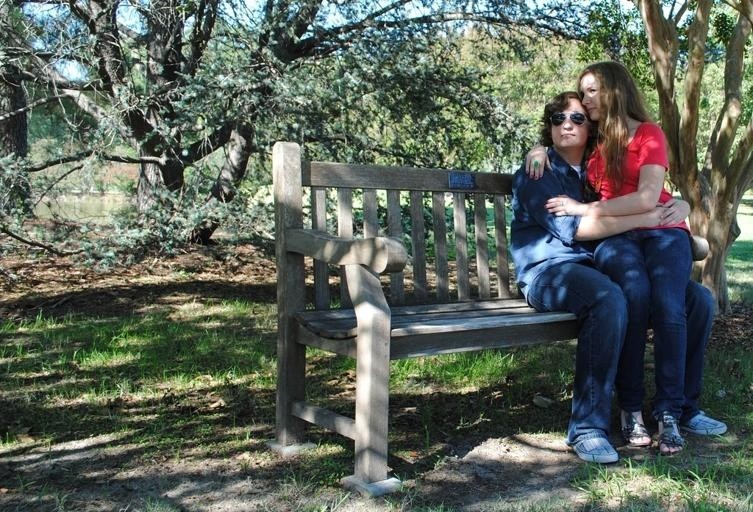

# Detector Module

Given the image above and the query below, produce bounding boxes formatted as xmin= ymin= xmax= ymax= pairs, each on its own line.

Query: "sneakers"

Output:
xmin=571 ymin=436 xmax=620 ymax=465
xmin=681 ymin=412 xmax=728 ymax=436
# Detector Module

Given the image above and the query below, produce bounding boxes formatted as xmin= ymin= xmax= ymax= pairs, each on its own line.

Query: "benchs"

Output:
xmin=268 ymin=137 xmax=712 ymax=497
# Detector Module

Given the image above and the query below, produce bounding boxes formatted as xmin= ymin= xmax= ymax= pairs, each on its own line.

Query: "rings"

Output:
xmin=533 ymin=159 xmax=540 ymax=168
xmin=561 ymin=201 xmax=564 ymax=206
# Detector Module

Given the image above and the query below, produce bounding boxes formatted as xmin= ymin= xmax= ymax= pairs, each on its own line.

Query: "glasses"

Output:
xmin=548 ymin=112 xmax=586 ymax=125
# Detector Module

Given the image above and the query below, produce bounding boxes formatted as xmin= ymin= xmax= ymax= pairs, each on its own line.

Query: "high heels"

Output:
xmin=659 ymin=416 xmax=685 ymax=456
xmin=620 ymin=408 xmax=653 ymax=446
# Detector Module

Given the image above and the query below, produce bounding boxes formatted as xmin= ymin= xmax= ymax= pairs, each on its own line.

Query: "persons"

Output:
xmin=526 ymin=61 xmax=693 ymax=456
xmin=510 ymin=91 xmax=728 ymax=463
